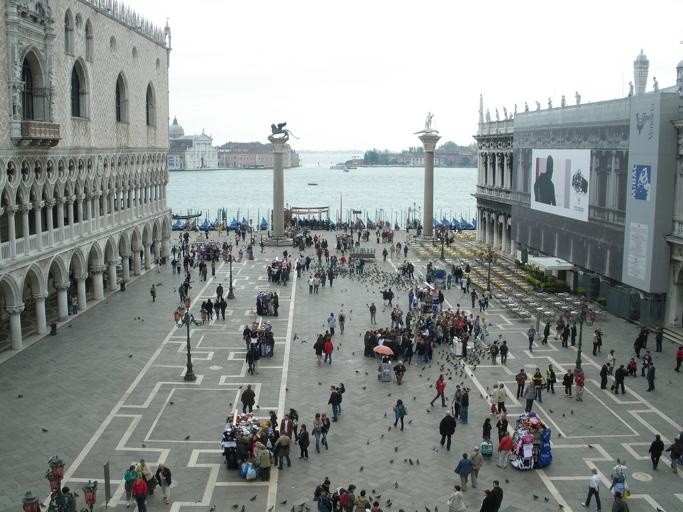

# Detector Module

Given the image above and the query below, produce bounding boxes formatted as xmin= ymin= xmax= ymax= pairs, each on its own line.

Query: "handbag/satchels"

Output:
xmin=312 ymin=429 xmax=315 ymax=435
xmin=269 ymin=451 xmax=275 ymax=464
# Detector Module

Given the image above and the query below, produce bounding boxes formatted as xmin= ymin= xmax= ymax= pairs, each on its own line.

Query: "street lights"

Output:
xmin=22 ymin=454 xmax=98 ymax=512
xmin=246 ymin=218 xmax=254 ymax=260
xmin=173 ymin=296 xmax=208 ymax=383
xmin=222 ymin=242 xmax=243 ymax=299
xmin=437 ymin=227 xmax=446 ymax=259
xmin=566 ymin=294 xmax=595 ymax=378
xmin=481 ymin=250 xmax=497 ymax=299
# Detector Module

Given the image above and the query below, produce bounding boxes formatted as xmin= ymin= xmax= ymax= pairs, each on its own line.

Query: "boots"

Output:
xmin=321 ymin=438 xmax=325 ymax=445
xmin=325 ymin=442 xmax=328 ymax=449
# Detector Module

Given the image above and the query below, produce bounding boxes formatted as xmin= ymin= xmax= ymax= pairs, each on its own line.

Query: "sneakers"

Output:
xmin=581 ymin=503 xmax=587 ymax=507
xmin=596 ymin=508 xmax=600 ymax=511
xmin=166 ymin=499 xmax=169 ymax=504
xmin=304 ymin=457 xmax=308 ymax=460
xmin=299 ymin=456 xmax=302 ymax=459
xmin=162 ymin=499 xmax=166 ymax=502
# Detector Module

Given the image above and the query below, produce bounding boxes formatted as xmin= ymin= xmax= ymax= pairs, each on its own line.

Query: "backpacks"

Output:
xmin=340 ymin=491 xmax=348 ymax=505
xmin=319 ymin=496 xmax=332 ymax=512
xmin=401 ymin=407 xmax=406 ymax=417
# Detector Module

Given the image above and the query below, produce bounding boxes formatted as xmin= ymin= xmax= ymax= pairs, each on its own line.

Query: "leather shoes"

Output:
xmin=278 ymin=466 xmax=283 ymax=469
xmin=332 ymin=419 xmax=337 ymax=422
xmin=496 ymin=464 xmax=502 ymax=468
xmin=317 ymin=449 xmax=320 ymax=453
xmin=504 ymin=466 xmax=507 ymax=469
xmin=332 ymin=417 xmax=335 ymax=418
xmin=127 ymin=502 xmax=132 ymax=507
xmin=288 ymin=462 xmax=291 ymax=466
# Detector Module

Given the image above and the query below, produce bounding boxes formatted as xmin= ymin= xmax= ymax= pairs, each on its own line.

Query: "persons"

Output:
xmin=652 ymin=76 xmax=658 ymax=91
xmin=534 ymin=155 xmax=557 ymax=206
xmin=426 ymin=112 xmax=432 ymax=128
xmin=628 ymin=81 xmax=633 ymax=97
xmin=485 ymin=91 xmax=582 ymax=123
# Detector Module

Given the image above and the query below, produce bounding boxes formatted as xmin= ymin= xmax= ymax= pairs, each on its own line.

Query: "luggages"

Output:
xmin=480 ymin=440 xmax=492 ymax=457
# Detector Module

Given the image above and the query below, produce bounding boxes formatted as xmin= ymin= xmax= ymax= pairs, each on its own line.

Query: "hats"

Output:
xmin=319 ymin=488 xmax=326 ymax=492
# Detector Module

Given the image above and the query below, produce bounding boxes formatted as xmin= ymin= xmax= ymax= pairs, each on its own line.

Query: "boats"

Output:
xmin=171 ymin=210 xmax=217 ymax=230
xmin=230 ymin=216 xmax=239 ymax=229
xmin=328 ymin=216 xmax=391 ymax=229
xmin=405 ymin=219 xmax=422 ymax=229
xmin=430 ymin=215 xmax=476 ymax=230
xmin=239 ymin=217 xmax=248 ymax=230
xmin=255 ymin=217 xmax=268 ymax=230
